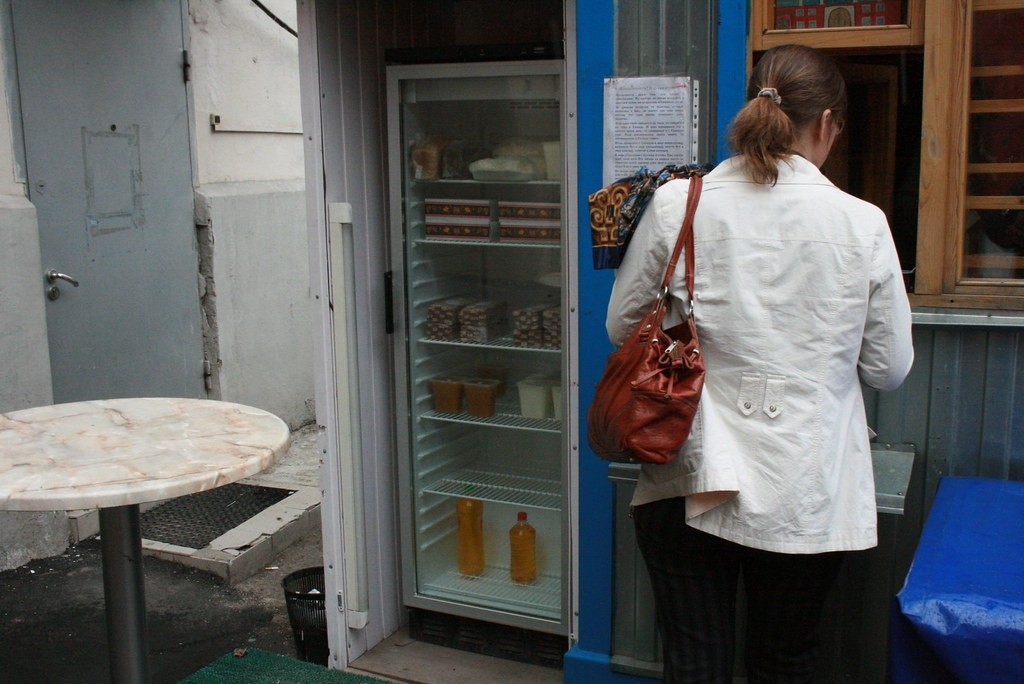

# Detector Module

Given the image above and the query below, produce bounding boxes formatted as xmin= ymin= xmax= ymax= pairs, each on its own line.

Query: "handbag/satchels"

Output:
xmin=588 ymin=174 xmax=705 ymax=465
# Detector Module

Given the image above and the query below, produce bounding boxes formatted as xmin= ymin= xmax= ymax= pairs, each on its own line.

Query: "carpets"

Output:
xmin=174 ymin=647 xmax=396 ymax=684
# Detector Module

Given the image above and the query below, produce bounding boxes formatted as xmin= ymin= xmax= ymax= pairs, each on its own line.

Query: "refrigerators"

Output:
xmin=382 ymin=55 xmax=575 ymax=651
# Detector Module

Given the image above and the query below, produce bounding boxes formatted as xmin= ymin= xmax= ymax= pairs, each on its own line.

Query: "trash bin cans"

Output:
xmin=280 ymin=565 xmax=329 ymax=669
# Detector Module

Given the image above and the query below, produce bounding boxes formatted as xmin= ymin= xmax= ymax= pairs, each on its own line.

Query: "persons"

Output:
xmin=606 ymin=45 xmax=914 ymax=684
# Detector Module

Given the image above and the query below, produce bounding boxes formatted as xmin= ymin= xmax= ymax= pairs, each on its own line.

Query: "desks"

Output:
xmin=0 ymin=396 xmax=292 ymax=684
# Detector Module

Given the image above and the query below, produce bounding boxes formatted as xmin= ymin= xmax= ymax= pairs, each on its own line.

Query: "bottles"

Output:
xmin=508 ymin=510 xmax=536 ymax=584
xmin=456 ymin=485 xmax=485 ymax=576
